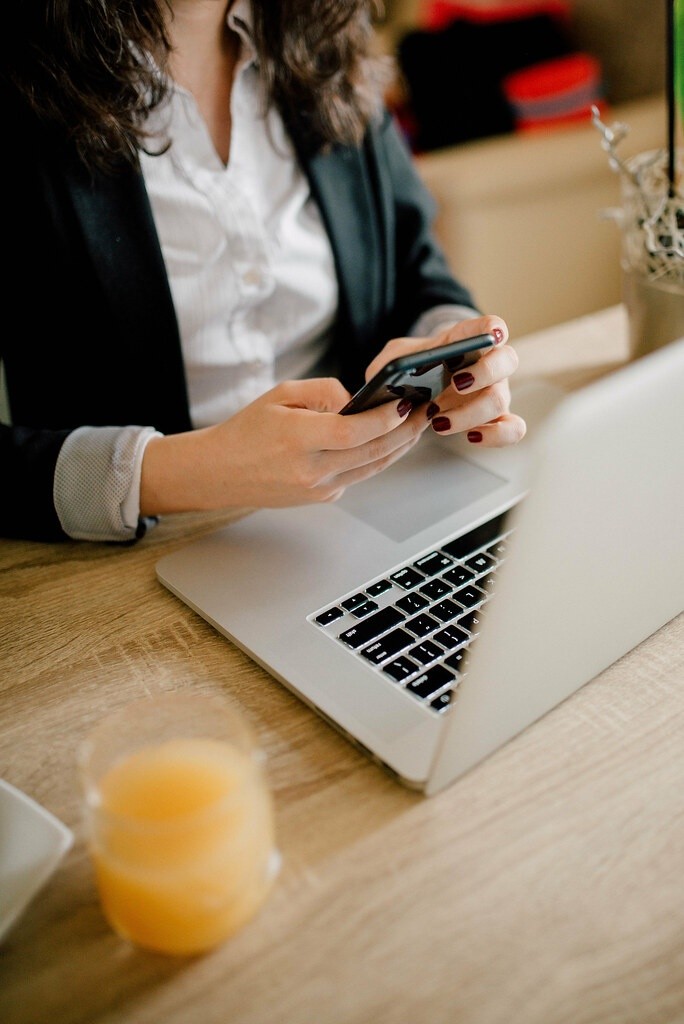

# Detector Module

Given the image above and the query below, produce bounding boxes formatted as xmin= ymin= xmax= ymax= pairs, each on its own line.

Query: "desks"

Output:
xmin=0 ymin=501 xmax=684 ymax=1024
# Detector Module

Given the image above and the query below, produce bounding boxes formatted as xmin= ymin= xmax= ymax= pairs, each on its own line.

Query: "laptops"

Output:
xmin=155 ymin=337 xmax=684 ymax=799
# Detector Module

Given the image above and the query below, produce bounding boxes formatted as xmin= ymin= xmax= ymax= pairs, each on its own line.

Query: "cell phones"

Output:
xmin=339 ymin=333 xmax=495 ymax=415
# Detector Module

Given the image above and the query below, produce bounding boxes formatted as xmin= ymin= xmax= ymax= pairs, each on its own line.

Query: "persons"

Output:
xmin=0 ymin=0 xmax=526 ymax=546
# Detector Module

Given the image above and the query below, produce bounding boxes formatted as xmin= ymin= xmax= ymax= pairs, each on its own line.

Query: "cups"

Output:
xmin=76 ymin=695 xmax=270 ymax=955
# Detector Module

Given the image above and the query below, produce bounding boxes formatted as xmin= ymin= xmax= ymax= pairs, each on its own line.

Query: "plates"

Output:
xmin=0 ymin=778 xmax=73 ymax=948
